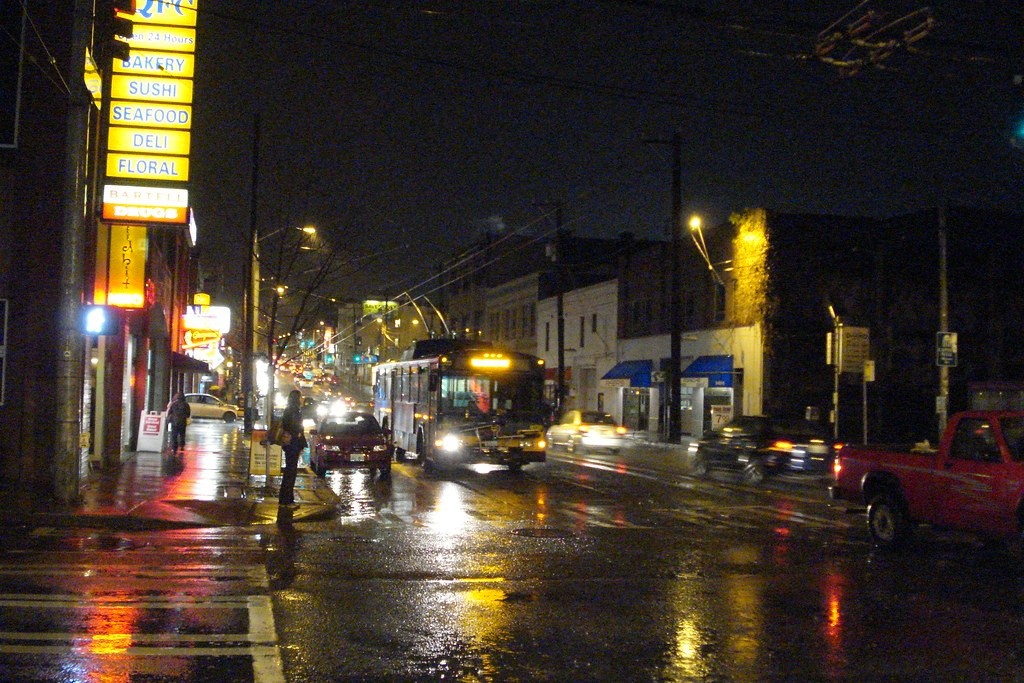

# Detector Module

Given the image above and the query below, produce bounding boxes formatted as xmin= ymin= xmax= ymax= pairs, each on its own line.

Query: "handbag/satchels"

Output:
xmin=269 ymin=426 xmax=291 ymax=447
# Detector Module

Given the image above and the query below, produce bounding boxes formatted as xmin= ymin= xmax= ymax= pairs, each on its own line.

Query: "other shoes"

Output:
xmin=181 ymin=447 xmax=184 ymax=451
xmin=279 ymin=501 xmax=300 ymax=508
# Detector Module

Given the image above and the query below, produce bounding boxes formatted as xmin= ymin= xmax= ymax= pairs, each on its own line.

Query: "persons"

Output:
xmin=279 ymin=390 xmax=309 ymax=508
xmin=166 ymin=392 xmax=190 ymax=454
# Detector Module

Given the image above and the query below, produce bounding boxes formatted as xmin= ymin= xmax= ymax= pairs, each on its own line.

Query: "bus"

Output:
xmin=370 ymin=292 xmax=560 ymax=473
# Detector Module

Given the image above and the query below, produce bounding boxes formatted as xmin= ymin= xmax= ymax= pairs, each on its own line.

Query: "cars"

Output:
xmin=184 ymin=392 xmax=239 ymax=422
xmin=273 ymin=359 xmax=357 ymax=420
xmin=545 ymin=408 xmax=633 ymax=455
xmin=307 ymin=411 xmax=393 ymax=478
xmin=692 ymin=415 xmax=837 ymax=490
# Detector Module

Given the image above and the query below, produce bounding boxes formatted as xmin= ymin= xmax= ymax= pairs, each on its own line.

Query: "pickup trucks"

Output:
xmin=826 ymin=408 xmax=1024 ymax=557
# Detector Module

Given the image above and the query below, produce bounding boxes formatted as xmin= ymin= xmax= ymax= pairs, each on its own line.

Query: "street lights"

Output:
xmin=250 ymin=223 xmax=316 ymax=433
xmin=531 ymin=196 xmax=566 ymax=424
xmin=640 ymin=130 xmax=684 ymax=444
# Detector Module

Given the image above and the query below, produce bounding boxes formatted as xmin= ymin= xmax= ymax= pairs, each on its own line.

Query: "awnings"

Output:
xmin=681 ymin=355 xmax=733 ymax=387
xmin=599 ymin=360 xmax=651 ymax=387
